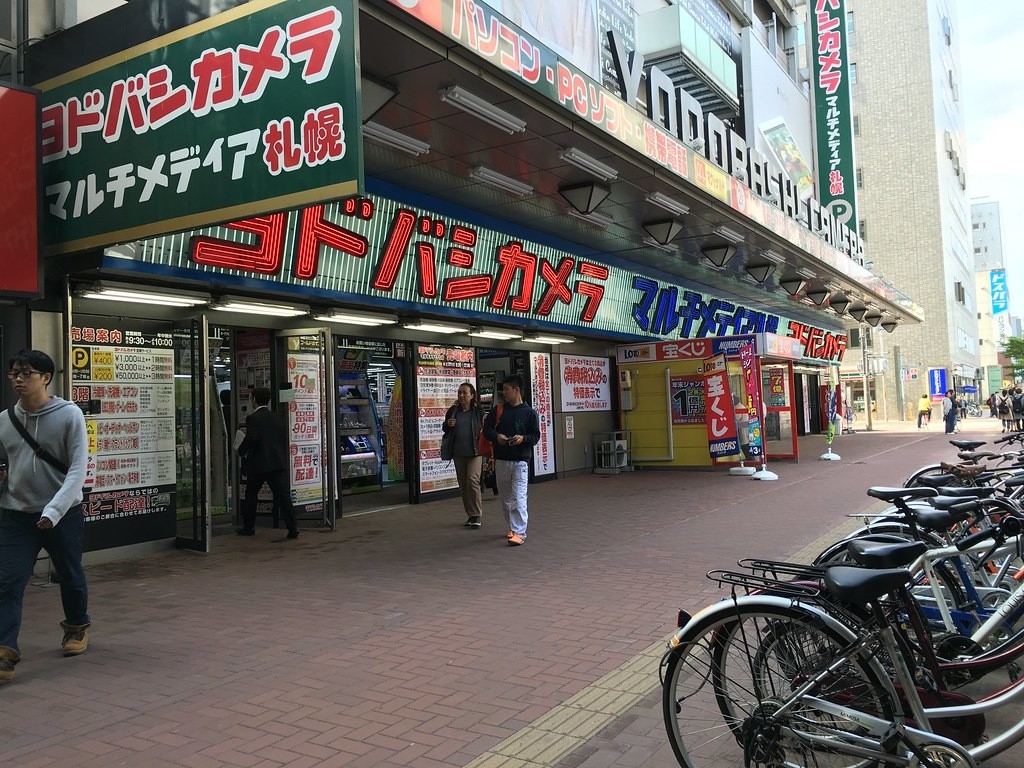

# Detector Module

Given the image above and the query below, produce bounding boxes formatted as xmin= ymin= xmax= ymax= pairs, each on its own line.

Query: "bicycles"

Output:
xmin=659 ymin=428 xmax=1024 ymax=768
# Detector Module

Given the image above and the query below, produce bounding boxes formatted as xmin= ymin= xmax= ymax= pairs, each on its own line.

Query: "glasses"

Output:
xmin=7 ymin=369 xmax=43 ymax=379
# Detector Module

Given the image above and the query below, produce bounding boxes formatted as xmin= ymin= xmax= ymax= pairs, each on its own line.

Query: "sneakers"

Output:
xmin=0 ymin=659 xmax=21 ymax=680
xmin=60 ymin=630 xmax=91 ymax=656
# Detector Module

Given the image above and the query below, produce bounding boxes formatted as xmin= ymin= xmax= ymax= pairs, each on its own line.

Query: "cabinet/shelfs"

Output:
xmin=339 ymin=372 xmax=382 ymax=490
xmin=479 ymin=369 xmax=505 ymax=413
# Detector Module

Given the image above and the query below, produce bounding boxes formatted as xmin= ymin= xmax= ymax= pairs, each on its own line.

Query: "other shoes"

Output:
xmin=506 ymin=530 xmax=515 ymax=538
xmin=464 ymin=517 xmax=472 ymax=526
xmin=471 ymin=516 xmax=482 ymax=527
xmin=508 ymin=535 xmax=525 ymax=544
xmin=286 ymin=524 xmax=300 ymax=538
xmin=235 ymin=527 xmax=256 ymax=536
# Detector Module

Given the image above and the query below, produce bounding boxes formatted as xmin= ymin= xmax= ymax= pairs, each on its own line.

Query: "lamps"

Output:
xmin=361 ymin=121 xmax=431 ymax=156
xmin=556 ymin=147 xmax=618 ymax=181
xmin=697 ymin=226 xmax=906 ymax=333
xmin=642 ymin=218 xmax=684 ymax=246
xmin=641 ymin=191 xmax=690 ymax=216
xmin=359 ymin=71 xmax=401 ymax=125
xmin=556 ymin=180 xmax=612 ymax=215
xmin=565 ymin=207 xmax=615 ymax=228
xmin=438 ymin=85 xmax=527 ymax=135
xmin=640 ymin=236 xmax=679 ymax=252
xmin=466 ymin=166 xmax=534 ymax=197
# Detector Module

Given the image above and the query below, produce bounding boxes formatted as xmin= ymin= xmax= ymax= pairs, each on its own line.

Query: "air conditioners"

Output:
xmin=602 ymin=440 xmax=627 ymax=468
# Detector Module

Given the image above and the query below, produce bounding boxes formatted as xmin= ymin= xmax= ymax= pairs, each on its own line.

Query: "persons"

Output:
xmin=440 ymin=383 xmax=485 ymax=526
xmin=986 ymin=388 xmax=1024 ymax=432
xmin=942 ymin=389 xmax=967 ymax=434
xmin=917 ymin=394 xmax=932 ymax=428
xmin=484 ymin=374 xmax=541 ymax=544
xmin=0 ymin=350 xmax=91 ymax=680
xmin=235 ymin=387 xmax=301 ymax=539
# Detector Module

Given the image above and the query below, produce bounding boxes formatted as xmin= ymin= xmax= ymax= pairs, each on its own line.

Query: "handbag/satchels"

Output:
xmin=440 ymin=432 xmax=454 ymax=469
xmin=476 ymin=404 xmax=503 ymax=458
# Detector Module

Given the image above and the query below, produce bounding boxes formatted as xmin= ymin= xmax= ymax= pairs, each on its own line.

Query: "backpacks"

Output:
xmin=998 ymin=397 xmax=1009 ymax=414
xmin=1012 ymin=395 xmax=1022 ymax=413
